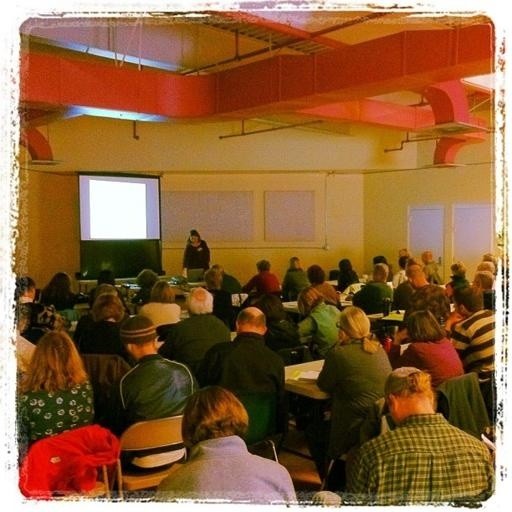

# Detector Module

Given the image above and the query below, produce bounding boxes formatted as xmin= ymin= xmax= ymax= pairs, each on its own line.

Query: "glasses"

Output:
xmin=336 ymin=321 xmax=343 ymax=329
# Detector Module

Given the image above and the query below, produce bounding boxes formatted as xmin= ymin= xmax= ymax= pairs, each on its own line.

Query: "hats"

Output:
xmin=380 ymin=366 xmax=422 ymax=416
xmin=119 ymin=315 xmax=157 ymax=344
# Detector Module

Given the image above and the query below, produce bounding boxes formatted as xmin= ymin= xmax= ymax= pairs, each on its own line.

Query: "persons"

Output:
xmin=13 ymin=248 xmax=498 ymax=502
xmin=181 ymin=230 xmax=211 ymax=281
